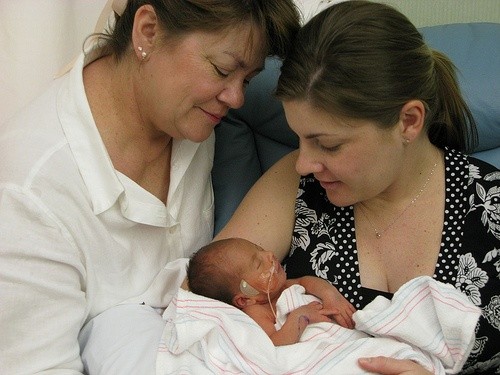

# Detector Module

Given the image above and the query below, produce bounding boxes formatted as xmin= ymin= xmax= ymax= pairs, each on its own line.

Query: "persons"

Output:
xmin=180 ymin=0 xmax=500 ymax=375
xmin=0 ymin=0 xmax=302 ymax=375
xmin=186 ymin=238 xmax=482 ymax=375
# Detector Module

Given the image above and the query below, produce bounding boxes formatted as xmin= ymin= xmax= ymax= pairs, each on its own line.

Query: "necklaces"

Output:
xmin=358 ymin=148 xmax=439 ymax=240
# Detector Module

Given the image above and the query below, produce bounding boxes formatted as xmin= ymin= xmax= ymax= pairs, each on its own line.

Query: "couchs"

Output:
xmin=213 ymin=22 xmax=500 ymax=241
